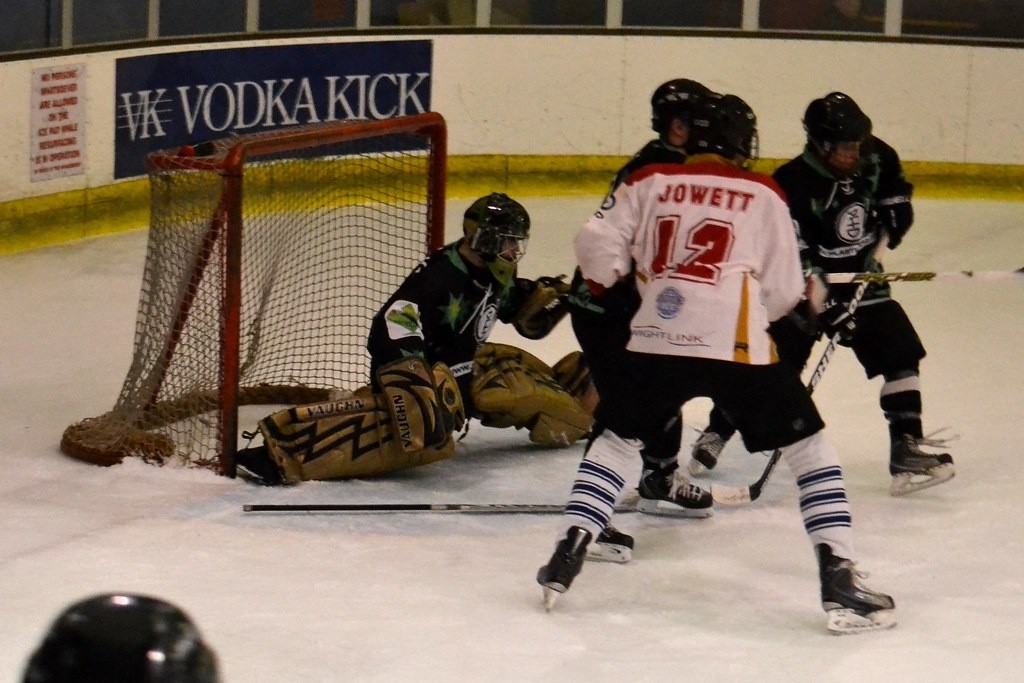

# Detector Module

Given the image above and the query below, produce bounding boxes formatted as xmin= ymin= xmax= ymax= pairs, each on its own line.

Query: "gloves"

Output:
xmin=866 ymin=202 xmax=913 ymax=249
xmin=816 ymin=303 xmax=856 ymax=347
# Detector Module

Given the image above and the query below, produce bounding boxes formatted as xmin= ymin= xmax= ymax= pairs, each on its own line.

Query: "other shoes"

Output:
xmin=234 ymin=446 xmax=282 ymax=486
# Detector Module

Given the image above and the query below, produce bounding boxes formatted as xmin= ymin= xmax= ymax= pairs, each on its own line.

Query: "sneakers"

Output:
xmin=636 ymin=450 xmax=715 ymax=518
xmin=535 ymin=526 xmax=591 ymax=612
xmin=585 ymin=523 xmax=634 ymax=563
xmin=686 ymin=424 xmax=726 ymax=475
xmin=888 ymin=425 xmax=955 ymax=496
xmin=813 ymin=542 xmax=898 ymax=632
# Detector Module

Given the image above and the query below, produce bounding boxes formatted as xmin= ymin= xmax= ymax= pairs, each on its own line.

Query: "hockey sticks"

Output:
xmin=801 ymin=263 xmax=1024 ymax=286
xmin=243 ymin=487 xmax=637 ymax=514
xmin=708 ymin=229 xmax=891 ymax=505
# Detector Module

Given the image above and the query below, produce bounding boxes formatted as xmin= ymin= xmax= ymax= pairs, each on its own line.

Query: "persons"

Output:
xmin=571 ymin=73 xmax=955 ymax=512
xmin=20 ymin=590 xmax=222 ymax=683
xmin=535 ymin=90 xmax=898 ymax=619
xmin=234 ymin=192 xmax=600 ymax=487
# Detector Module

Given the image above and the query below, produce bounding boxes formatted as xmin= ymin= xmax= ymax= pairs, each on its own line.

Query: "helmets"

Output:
xmin=462 ymin=192 xmax=531 ymax=263
xmin=801 ymin=92 xmax=872 ymax=146
xmin=684 ymin=94 xmax=756 ymax=160
xmin=651 ymin=77 xmax=711 ymax=135
xmin=19 ymin=593 xmax=218 ymax=683
